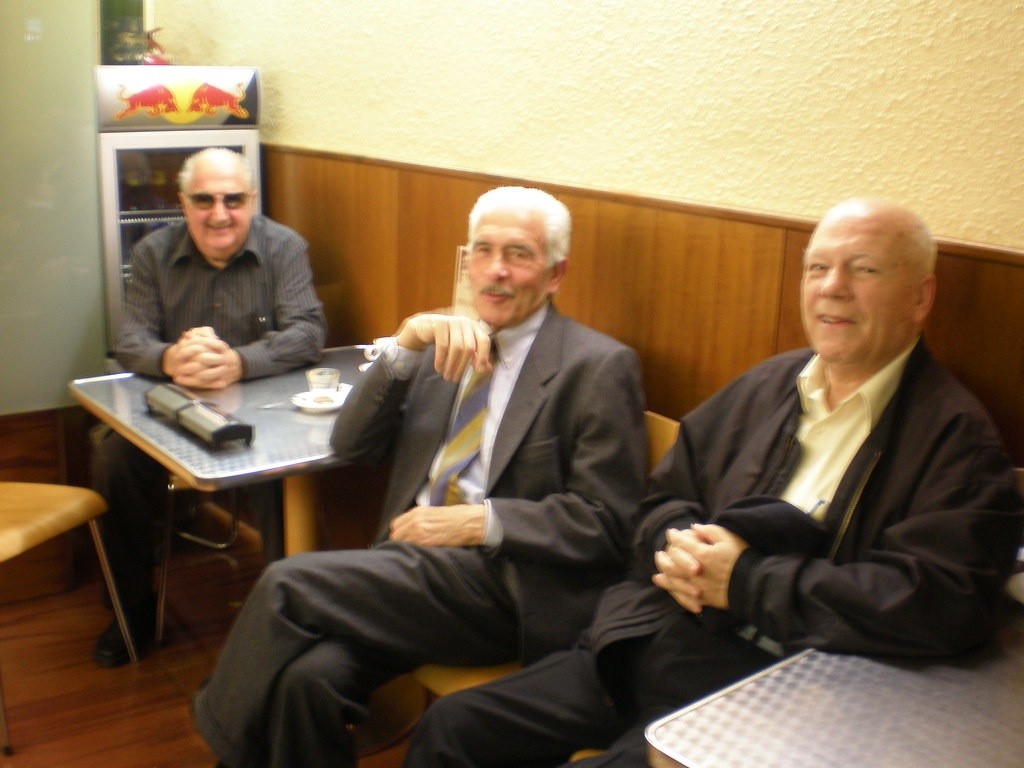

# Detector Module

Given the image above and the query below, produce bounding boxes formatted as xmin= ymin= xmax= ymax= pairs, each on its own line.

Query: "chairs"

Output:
xmin=0 ymin=409 xmax=1024 ymax=761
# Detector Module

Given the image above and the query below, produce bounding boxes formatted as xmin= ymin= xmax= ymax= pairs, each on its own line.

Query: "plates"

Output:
xmin=293 ymin=391 xmax=347 ymax=412
xmin=359 ymin=362 xmax=374 ymax=373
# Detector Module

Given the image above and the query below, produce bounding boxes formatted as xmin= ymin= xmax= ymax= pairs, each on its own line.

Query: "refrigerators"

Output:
xmin=94 ymin=64 xmax=260 ymax=360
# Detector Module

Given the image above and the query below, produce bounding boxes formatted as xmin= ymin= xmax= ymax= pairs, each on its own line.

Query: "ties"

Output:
xmin=430 ymin=340 xmax=499 ymax=507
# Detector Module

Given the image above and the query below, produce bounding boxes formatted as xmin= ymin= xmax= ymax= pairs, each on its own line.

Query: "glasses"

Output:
xmin=182 ymin=191 xmax=260 ymax=211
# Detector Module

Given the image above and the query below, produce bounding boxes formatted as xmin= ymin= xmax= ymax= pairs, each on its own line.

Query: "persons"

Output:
xmin=403 ymin=201 xmax=1024 ymax=768
xmin=194 ymin=186 xmax=650 ymax=768
xmin=92 ymin=148 xmax=326 ymax=666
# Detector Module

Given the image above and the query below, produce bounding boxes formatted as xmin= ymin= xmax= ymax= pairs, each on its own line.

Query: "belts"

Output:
xmin=734 ymin=622 xmax=784 ymax=655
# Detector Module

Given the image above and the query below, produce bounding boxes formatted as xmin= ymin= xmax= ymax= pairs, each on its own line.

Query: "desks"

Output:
xmin=68 ymin=347 xmax=371 ymax=561
xmin=645 ymin=566 xmax=1024 ymax=768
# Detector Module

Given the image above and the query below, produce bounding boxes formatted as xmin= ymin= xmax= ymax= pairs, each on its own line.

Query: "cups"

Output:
xmin=364 ymin=337 xmax=392 ymax=360
xmin=306 ymin=368 xmax=340 ymax=404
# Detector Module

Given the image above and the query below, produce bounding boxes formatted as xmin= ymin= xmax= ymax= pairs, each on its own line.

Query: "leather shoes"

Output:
xmin=96 ymin=608 xmax=158 ymax=667
